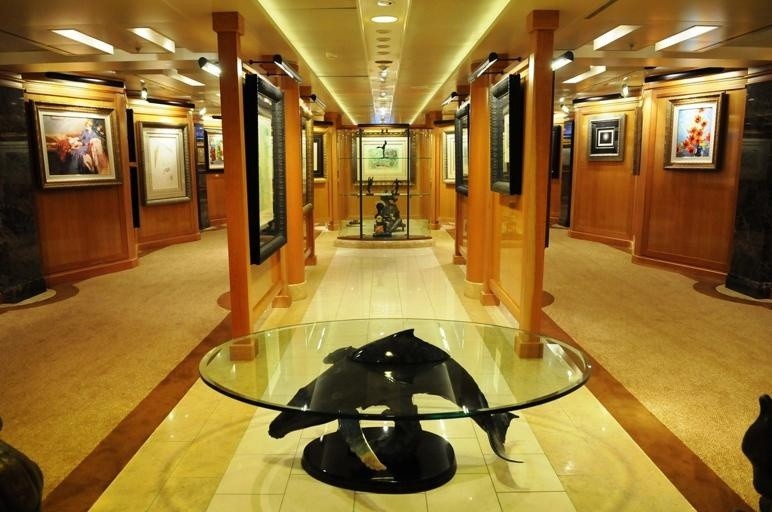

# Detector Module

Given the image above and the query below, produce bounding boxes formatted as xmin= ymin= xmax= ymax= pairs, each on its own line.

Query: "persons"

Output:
xmin=366 ymin=177 xmax=374 ymax=194
xmin=376 ymin=140 xmax=387 ymax=158
xmin=374 ymin=198 xmax=406 ymax=231
xmin=93 ymin=123 xmax=105 ymax=138
xmin=390 ymin=179 xmax=402 ymax=193
xmin=78 ymin=121 xmax=104 ymax=147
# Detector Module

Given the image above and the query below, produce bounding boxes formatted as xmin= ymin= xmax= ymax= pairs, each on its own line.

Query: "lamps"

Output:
xmin=558 ymin=101 xmax=575 ymax=115
xmin=620 ymin=76 xmax=644 ymax=100
xmin=548 ymin=50 xmax=576 ymax=74
xmin=198 ymin=55 xmax=222 ymax=78
xmin=243 ymin=52 xmax=305 ymax=84
xmin=122 ymin=86 xmax=148 ymax=102
xmin=299 ymin=91 xmax=327 ymax=113
xmin=466 ymin=51 xmax=525 ymax=85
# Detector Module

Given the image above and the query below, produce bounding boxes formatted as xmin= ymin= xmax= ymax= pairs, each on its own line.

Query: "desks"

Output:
xmin=192 ymin=317 xmax=595 ymax=496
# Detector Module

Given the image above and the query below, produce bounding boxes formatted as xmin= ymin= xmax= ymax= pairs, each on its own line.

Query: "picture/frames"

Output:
xmin=311 ymin=134 xmax=324 ymax=179
xmin=435 ymin=71 xmax=566 ymax=201
xmin=233 ymin=71 xmax=289 ymax=266
xmin=346 ymin=129 xmax=421 ymax=191
xmin=202 ymin=128 xmax=226 ymax=175
xmin=585 ymin=111 xmax=628 ymax=163
xmin=660 ymin=89 xmax=728 ymax=173
xmin=134 ymin=118 xmax=194 ymax=209
xmin=27 ymin=96 xmax=126 ymax=190
